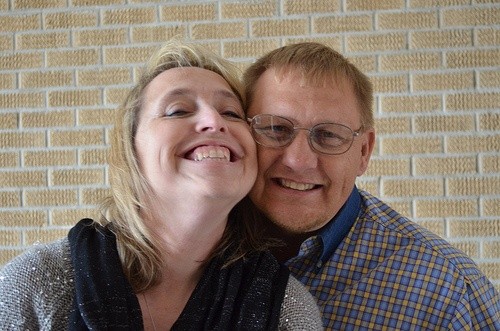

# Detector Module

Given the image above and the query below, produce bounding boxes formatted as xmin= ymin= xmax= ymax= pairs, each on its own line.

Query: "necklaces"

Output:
xmin=142 ymin=284 xmax=194 ymax=331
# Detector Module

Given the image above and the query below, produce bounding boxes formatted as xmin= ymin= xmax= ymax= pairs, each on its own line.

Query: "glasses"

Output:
xmin=246 ymin=112 xmax=369 ymax=156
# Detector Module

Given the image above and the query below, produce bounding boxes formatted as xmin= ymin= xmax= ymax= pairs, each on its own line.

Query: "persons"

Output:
xmin=0 ymin=39 xmax=323 ymax=331
xmin=241 ymin=43 xmax=500 ymax=331
